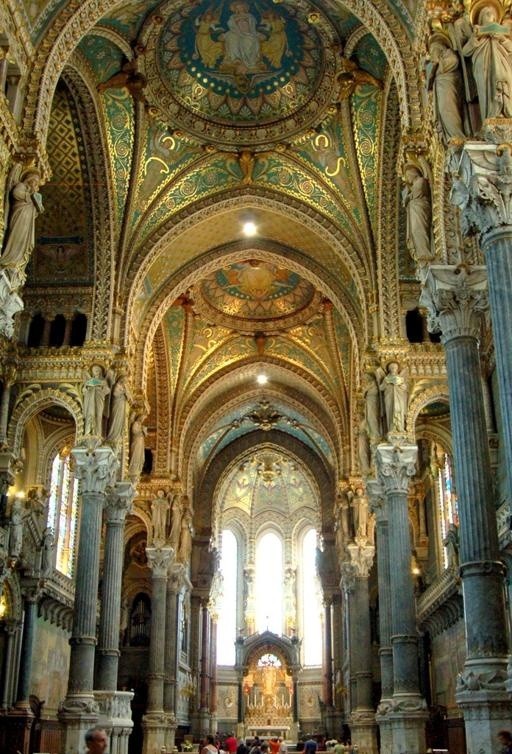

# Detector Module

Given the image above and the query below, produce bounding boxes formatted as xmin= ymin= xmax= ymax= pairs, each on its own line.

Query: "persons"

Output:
xmin=170 ymin=493 xmax=185 ymax=548
xmin=126 ymin=409 xmax=148 ymax=487
xmin=149 ymin=488 xmax=169 ymax=545
xmin=83 ymin=726 xmax=110 ymax=753
xmin=400 ymin=162 xmax=434 ymax=261
xmin=0 ymin=164 xmax=46 ymax=284
xmin=179 ymin=512 xmax=193 ymax=559
xmin=496 ymin=729 xmax=511 ymax=754
xmin=425 ymin=30 xmax=472 ymax=148
xmin=10 ymin=502 xmax=29 ymax=556
xmin=377 ymin=356 xmax=410 ymax=438
xmin=258 ymin=5 xmax=287 ymax=68
xmin=200 ymin=726 xmax=354 ymax=753
xmin=79 ymin=359 xmax=113 ymax=438
xmin=194 ymin=10 xmax=227 ymax=69
xmin=348 ymin=488 xmax=371 ymax=540
xmin=459 ymin=0 xmax=511 ymax=120
xmin=220 ymin=0 xmax=266 ymax=70
